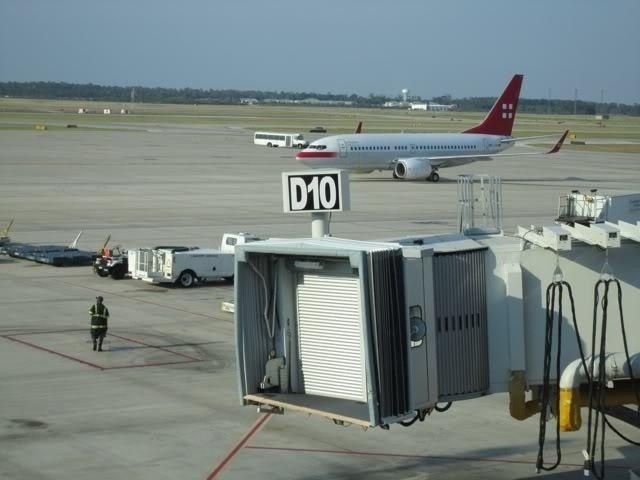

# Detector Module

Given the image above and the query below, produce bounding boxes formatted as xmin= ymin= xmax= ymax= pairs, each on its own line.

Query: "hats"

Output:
xmin=96 ymin=296 xmax=103 ymax=301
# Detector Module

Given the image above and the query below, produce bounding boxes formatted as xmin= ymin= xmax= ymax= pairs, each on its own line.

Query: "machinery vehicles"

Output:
xmin=125 ymin=232 xmax=270 ymax=287
xmin=89 ymin=235 xmax=201 ymax=280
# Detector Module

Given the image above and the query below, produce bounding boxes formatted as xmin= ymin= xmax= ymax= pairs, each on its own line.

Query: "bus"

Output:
xmin=253 ymin=131 xmax=309 ymax=149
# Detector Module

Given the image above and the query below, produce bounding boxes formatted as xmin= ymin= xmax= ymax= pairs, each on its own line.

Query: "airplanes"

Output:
xmin=295 ymin=73 xmax=571 ymax=181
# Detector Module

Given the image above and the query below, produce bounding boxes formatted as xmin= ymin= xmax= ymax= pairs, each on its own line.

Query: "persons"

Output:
xmin=88 ymin=296 xmax=110 ymax=352
